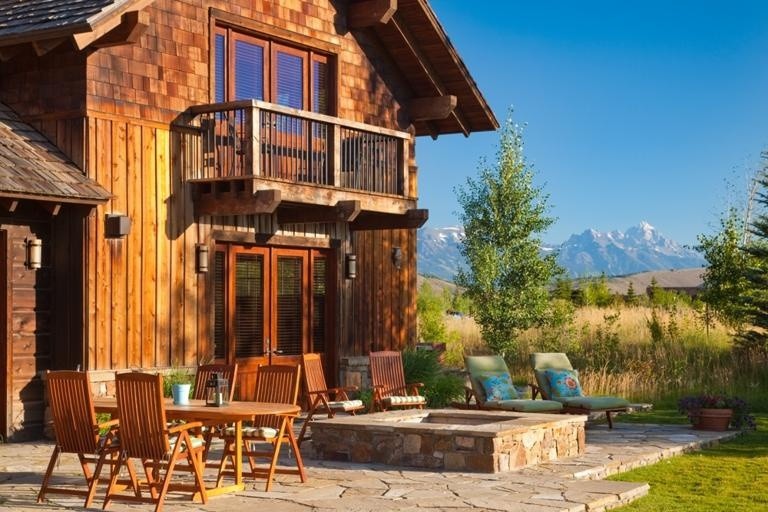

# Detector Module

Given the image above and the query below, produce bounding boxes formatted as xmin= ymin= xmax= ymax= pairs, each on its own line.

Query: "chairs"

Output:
xmin=296 ymin=352 xmax=365 ymax=449
xmin=179 ymin=363 xmax=243 ymax=481
xmin=529 ymin=352 xmax=631 ymax=429
xmin=369 ymin=351 xmax=427 ymax=412
xmin=103 ymin=369 xmax=210 ymax=512
xmin=461 ymin=354 xmax=565 ymax=415
xmin=215 ymin=363 xmax=307 ymax=492
xmin=36 ymin=369 xmax=145 ymax=508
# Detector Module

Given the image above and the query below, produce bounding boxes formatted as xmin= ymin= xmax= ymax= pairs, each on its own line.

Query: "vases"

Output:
xmin=688 ymin=408 xmax=734 ymax=432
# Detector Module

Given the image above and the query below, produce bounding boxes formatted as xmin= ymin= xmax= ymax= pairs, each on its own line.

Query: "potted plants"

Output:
xmin=171 ymin=365 xmax=192 ymax=404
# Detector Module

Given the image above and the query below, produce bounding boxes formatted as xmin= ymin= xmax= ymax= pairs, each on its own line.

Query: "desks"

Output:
xmin=90 ymin=393 xmax=301 ymax=502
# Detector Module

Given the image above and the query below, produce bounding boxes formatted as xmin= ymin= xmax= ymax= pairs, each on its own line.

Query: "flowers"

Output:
xmin=678 ymin=393 xmax=757 ymax=432
